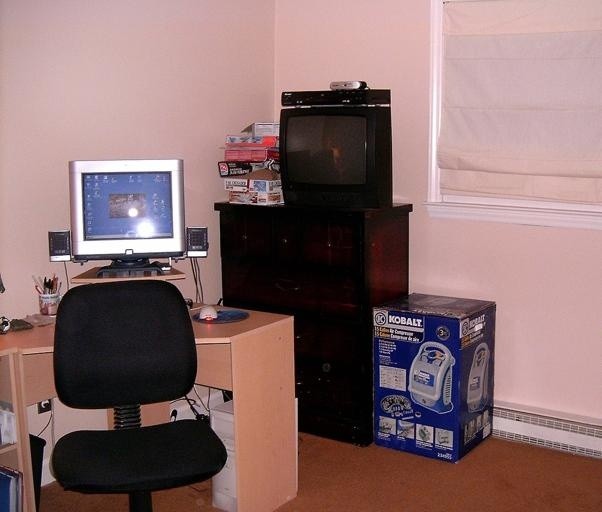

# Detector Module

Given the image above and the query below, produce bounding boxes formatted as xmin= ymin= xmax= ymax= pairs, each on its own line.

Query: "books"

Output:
xmin=0 ymin=466 xmax=24 ymax=512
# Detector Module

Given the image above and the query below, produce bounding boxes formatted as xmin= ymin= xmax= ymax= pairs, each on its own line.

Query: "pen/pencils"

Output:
xmin=32 ymin=274 xmax=61 ymax=294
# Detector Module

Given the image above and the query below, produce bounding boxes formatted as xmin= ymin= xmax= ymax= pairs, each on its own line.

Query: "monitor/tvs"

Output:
xmin=279 ymin=106 xmax=393 ymax=208
xmin=68 ymin=158 xmax=186 ymax=277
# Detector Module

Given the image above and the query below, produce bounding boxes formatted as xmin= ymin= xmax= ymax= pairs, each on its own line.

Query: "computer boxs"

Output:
xmin=209 ymin=396 xmax=300 ymax=512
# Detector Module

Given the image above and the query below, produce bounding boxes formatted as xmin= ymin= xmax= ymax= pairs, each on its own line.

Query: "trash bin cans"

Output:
xmin=29 ymin=433 xmax=46 ymax=512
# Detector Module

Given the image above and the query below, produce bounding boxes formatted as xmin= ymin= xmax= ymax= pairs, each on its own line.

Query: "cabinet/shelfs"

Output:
xmin=214 ymin=199 xmax=413 ymax=448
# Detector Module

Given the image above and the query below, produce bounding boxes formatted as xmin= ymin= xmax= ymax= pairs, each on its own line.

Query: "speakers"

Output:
xmin=186 ymin=227 xmax=208 ymax=257
xmin=47 ymin=229 xmax=71 ymax=262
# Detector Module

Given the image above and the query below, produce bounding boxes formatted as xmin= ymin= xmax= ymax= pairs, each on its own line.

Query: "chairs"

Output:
xmin=51 ymin=281 xmax=227 ymax=512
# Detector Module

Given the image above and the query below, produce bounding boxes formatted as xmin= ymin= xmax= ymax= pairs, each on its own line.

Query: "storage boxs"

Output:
xmin=372 ymin=292 xmax=497 ymax=465
xmin=224 ymin=123 xmax=281 ymax=163
xmin=224 ymin=178 xmax=283 ymax=206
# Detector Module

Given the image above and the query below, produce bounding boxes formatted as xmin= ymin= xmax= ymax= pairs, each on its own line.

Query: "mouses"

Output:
xmin=199 ymin=306 xmax=219 ymax=322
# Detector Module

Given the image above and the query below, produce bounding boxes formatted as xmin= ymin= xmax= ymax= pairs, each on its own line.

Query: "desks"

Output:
xmin=0 ymin=302 xmax=299 ymax=512
